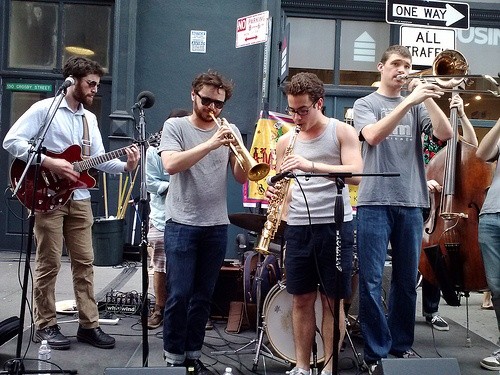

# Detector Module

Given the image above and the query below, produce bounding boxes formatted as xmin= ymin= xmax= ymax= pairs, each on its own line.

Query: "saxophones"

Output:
xmin=253 ymin=123 xmax=302 ymax=255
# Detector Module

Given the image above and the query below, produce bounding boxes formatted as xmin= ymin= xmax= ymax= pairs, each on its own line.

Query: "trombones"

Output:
xmin=396 ymin=49 xmax=500 ymax=97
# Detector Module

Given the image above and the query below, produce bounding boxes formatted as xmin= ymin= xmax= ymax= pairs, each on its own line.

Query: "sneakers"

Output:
xmin=206 ymin=319 xmax=214 ymax=329
xmin=186 ymin=359 xmax=214 ymax=375
xmin=148 ymin=311 xmax=163 ymax=328
xmin=480 ymin=352 xmax=500 ymax=370
xmin=426 ymin=315 xmax=449 ymax=330
xmin=361 ymin=359 xmax=378 ymax=375
xmin=400 ymin=350 xmax=415 ymax=358
xmin=167 ymin=362 xmax=184 ymax=366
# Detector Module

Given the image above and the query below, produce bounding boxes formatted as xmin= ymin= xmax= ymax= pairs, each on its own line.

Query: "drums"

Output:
xmin=262 ymin=280 xmax=347 ymax=365
xmin=335 ymin=229 xmax=359 ymax=274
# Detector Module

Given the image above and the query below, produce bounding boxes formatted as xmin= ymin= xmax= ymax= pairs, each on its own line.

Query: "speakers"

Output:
xmin=103 ymin=367 xmax=188 ymax=375
xmin=210 ymin=265 xmax=243 ymax=320
xmin=373 ymin=357 xmax=462 ymax=375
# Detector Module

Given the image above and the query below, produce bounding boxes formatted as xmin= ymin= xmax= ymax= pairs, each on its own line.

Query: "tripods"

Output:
xmin=0 ymin=87 xmax=77 ymax=375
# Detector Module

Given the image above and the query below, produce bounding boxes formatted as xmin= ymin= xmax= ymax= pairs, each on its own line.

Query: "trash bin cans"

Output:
xmin=92 ymin=215 xmax=126 ymax=267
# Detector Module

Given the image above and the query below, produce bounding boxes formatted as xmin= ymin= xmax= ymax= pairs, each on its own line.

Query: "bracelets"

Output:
xmin=311 ymin=162 xmax=315 ymax=174
xmin=458 ymin=112 xmax=466 ymax=117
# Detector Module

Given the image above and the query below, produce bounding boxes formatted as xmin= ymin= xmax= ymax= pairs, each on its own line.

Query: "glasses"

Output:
xmin=286 ymin=99 xmax=317 ymax=115
xmin=81 ymin=78 xmax=102 ymax=89
xmin=196 ymin=92 xmax=226 ymax=109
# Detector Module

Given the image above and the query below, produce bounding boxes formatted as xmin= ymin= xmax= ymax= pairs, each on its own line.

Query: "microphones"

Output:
xmin=266 ymin=171 xmax=290 ymax=186
xmin=59 ymin=77 xmax=75 ymax=91
xmin=132 ymin=91 xmax=155 ymax=111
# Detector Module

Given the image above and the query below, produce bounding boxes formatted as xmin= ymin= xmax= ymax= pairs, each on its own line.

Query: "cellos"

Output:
xmin=417 ymin=77 xmax=498 ymax=348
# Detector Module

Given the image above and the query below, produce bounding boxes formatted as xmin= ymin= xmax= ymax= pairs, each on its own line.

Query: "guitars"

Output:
xmin=9 ymin=130 xmax=165 ymax=213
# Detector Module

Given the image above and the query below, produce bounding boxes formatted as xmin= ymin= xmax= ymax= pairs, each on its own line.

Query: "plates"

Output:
xmin=55 ymin=299 xmax=98 ymax=313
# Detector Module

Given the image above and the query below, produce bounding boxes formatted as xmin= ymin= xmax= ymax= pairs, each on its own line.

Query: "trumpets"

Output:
xmin=208 ymin=110 xmax=270 ymax=182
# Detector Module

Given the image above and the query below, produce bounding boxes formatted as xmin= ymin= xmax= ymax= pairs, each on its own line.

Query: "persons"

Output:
xmin=145 ymin=109 xmax=194 ymax=328
xmin=353 ymin=46 xmax=454 ymax=375
xmin=474 ymin=118 xmax=500 ymax=370
xmin=250 ymin=184 xmax=292 ymax=303
xmin=419 ymin=94 xmax=478 ymax=331
xmin=342 ymin=273 xmax=357 ymax=317
xmin=265 ymin=72 xmax=364 ymax=375
xmin=2 ymin=55 xmax=141 ymax=352
xmin=156 ymin=67 xmax=249 ymax=375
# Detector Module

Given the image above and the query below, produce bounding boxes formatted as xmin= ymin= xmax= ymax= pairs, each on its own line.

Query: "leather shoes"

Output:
xmin=77 ymin=326 xmax=115 ymax=347
xmin=35 ymin=324 xmax=70 ymax=349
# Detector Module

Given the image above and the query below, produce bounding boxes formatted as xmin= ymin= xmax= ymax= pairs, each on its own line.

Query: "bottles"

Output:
xmin=223 ymin=367 xmax=234 ymax=375
xmin=38 ymin=340 xmax=52 ymax=375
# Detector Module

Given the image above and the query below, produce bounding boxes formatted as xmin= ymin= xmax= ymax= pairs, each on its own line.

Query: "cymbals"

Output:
xmin=228 ymin=213 xmax=288 ymax=240
